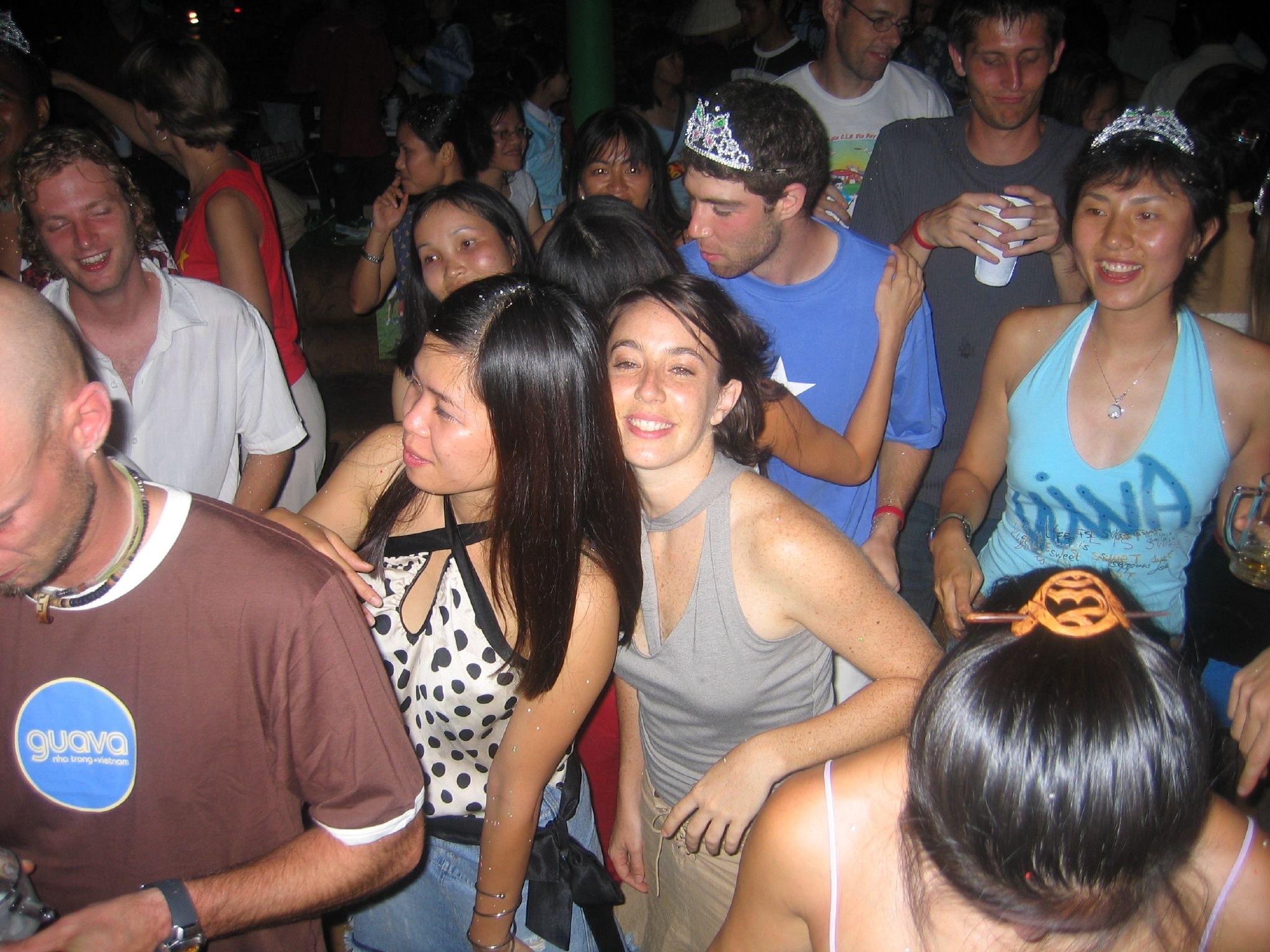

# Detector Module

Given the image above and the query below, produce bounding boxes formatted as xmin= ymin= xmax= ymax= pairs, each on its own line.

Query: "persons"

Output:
xmin=0 ymin=0 xmax=1270 ymax=655
xmin=392 ymin=179 xmax=625 ymax=897
xmin=606 ymin=273 xmax=949 ymax=952
xmin=0 ymin=274 xmax=426 ymax=952
xmin=262 ymin=273 xmax=641 ymax=952
xmin=1227 ymin=644 xmax=1270 ymax=797
xmin=704 ymin=567 xmax=1270 ymax=952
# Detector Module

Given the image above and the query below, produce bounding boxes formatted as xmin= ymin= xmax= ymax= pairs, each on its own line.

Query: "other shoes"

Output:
xmin=328 ymin=217 xmax=373 ymax=247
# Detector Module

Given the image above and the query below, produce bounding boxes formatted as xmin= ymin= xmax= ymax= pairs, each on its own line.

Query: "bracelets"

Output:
xmin=871 ymin=505 xmax=906 ymax=529
xmin=467 ymin=883 xmax=522 ymax=952
xmin=362 ymin=249 xmax=383 ymax=263
xmin=913 ymin=211 xmax=935 ymax=250
xmin=926 ymin=512 xmax=973 ymax=546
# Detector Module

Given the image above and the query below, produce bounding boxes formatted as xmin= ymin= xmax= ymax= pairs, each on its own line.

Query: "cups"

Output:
xmin=975 ymin=196 xmax=1033 ymax=288
xmin=0 ymin=847 xmax=56 ymax=943
xmin=1222 ymin=473 xmax=1270 ymax=590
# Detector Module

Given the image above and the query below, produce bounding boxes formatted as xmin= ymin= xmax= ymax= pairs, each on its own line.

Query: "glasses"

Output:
xmin=845 ymin=0 xmax=916 ymax=34
xmin=491 ymin=127 xmax=534 ymax=142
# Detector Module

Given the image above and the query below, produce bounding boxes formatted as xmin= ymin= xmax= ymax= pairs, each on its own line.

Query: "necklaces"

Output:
xmin=180 ymin=149 xmax=234 ymax=209
xmin=23 ymin=457 xmax=149 ymax=626
xmin=1088 ymin=316 xmax=1178 ymax=420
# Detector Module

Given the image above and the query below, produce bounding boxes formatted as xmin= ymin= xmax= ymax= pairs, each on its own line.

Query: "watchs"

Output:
xmin=139 ymin=878 xmax=207 ymax=952
